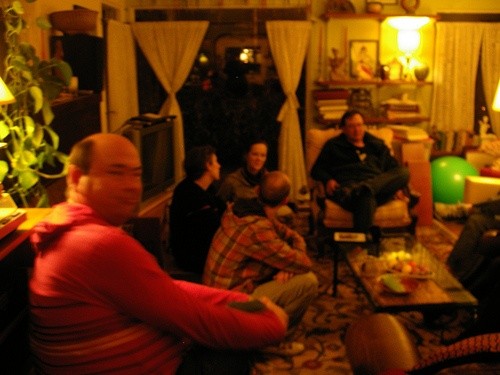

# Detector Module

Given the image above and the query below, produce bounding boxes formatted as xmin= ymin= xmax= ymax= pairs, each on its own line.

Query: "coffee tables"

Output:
xmin=332 ymin=233 xmax=479 ymax=326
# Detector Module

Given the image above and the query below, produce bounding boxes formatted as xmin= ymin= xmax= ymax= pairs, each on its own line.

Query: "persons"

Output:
xmin=29 ymin=133 xmax=289 ymax=375
xmin=447 ymin=200 xmax=500 ymax=321
xmin=167 ymin=62 xmax=330 ymax=355
xmin=309 ymin=109 xmax=410 ymax=248
xmin=352 ymin=46 xmax=376 ymax=80
xmin=329 ymin=48 xmax=347 ymax=82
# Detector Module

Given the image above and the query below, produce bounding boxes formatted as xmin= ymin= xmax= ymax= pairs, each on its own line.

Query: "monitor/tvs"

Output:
xmin=123 ymin=119 xmax=176 ymax=203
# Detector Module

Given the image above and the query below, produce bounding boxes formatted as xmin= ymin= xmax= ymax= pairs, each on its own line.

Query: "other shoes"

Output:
xmin=345 ymin=185 xmax=369 ymax=201
xmin=259 ymin=341 xmax=300 ymax=356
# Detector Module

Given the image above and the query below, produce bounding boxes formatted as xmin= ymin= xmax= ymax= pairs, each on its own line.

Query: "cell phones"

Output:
xmin=334 ymin=232 xmax=366 ymax=242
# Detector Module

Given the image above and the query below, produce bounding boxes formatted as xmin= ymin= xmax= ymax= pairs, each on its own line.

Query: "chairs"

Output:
xmin=306 ymin=127 xmax=419 ymax=257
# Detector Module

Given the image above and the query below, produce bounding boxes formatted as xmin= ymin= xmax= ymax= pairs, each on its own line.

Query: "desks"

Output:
xmin=0 ymin=208 xmax=50 ymax=375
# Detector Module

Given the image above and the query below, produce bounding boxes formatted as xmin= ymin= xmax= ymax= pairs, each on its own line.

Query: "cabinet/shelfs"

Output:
xmin=317 ymin=81 xmax=433 ymax=124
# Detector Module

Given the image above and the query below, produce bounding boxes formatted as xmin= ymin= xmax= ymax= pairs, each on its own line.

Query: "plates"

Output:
xmin=375 ymin=274 xmax=418 ymax=295
xmin=383 ymin=257 xmax=436 ymax=280
